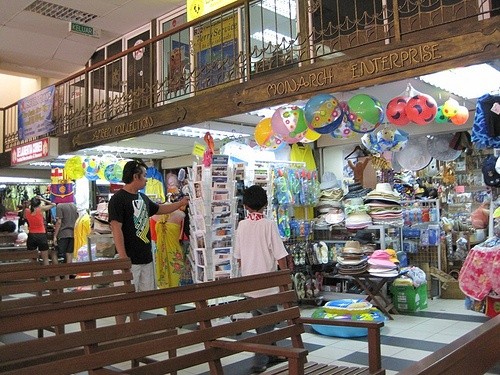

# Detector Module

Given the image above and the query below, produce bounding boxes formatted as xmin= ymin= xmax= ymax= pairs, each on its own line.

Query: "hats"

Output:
xmin=320 ymin=172 xmax=340 ymax=190
xmin=16 ymin=233 xmax=28 ymax=243
xmin=316 ymin=188 xmax=346 ymax=230
xmin=344 ymin=199 xmax=372 ymax=230
xmin=338 ymin=241 xmax=373 ymax=274
xmin=344 ymin=182 xmax=370 ymax=199
xmin=367 ymin=250 xmax=400 ymax=278
xmin=366 ymin=183 xmax=403 ymax=227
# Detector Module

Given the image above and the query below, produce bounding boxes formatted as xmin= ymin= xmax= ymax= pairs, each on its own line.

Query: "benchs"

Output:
xmin=0 ymin=250 xmax=385 ymax=375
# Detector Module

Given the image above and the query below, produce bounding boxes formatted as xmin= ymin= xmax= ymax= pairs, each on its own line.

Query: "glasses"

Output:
xmin=135 ymin=158 xmax=142 ymax=168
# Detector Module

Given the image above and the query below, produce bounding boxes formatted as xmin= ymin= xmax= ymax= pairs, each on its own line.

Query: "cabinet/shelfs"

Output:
xmin=253 ymin=159 xmax=500 ymax=298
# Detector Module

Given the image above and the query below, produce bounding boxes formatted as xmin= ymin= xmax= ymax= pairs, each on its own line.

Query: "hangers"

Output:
xmin=344 ymin=145 xmax=392 ymax=169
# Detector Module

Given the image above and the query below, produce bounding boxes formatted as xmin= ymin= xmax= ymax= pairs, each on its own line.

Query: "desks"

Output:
xmin=341 ymin=266 xmax=404 ymax=320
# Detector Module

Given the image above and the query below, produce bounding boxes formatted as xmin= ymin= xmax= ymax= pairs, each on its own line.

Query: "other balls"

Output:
xmin=255 ymin=94 xmax=384 ymax=152
xmin=98 ymin=154 xmax=134 ymax=183
xmin=386 ymin=94 xmax=469 ymax=126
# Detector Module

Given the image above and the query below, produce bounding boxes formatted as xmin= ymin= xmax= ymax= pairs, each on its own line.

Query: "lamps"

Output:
xmin=415 ymin=63 xmax=500 ymax=100
xmin=248 ymin=104 xmax=289 ymax=118
xmin=160 ymin=126 xmax=250 ymax=141
xmin=29 ymin=145 xmax=164 ymax=167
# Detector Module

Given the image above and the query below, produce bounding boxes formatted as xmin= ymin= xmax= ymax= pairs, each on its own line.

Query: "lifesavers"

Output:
xmin=75 ymin=244 xmax=96 ymax=291
xmin=361 ymin=130 xmax=408 ymax=153
xmin=311 ymin=307 xmax=386 ymax=337
xmin=83 ymin=156 xmax=101 ymax=176
xmin=323 ymin=298 xmax=372 ymax=315
xmin=84 ymin=173 xmax=99 ymax=181
xmin=65 ymin=157 xmax=84 ymax=179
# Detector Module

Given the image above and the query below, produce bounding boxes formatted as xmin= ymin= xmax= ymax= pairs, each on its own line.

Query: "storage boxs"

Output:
xmin=471 ymin=248 xmax=500 ymax=317
xmin=441 ymin=281 xmax=467 ymax=299
xmin=389 ymin=280 xmax=428 ymax=313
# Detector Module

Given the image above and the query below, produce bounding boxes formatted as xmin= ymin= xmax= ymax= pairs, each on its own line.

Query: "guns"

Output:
xmin=273 ymin=168 xmax=317 ymax=238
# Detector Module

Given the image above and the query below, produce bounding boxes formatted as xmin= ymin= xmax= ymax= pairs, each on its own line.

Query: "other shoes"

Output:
xmin=70 ymin=274 xmax=75 ymax=280
xmin=60 ymin=276 xmax=65 ymax=280
xmin=252 ymin=365 xmax=266 ymax=373
xmin=268 ymin=357 xmax=287 ymax=363
